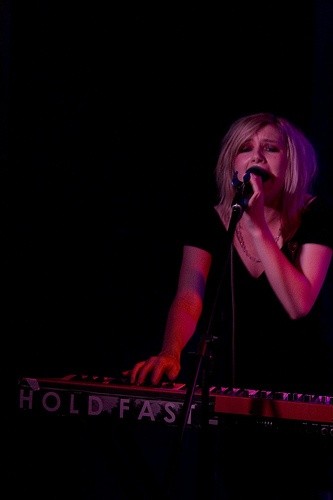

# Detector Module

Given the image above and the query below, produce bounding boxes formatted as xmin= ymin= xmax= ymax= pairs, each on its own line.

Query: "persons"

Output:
xmin=122 ymin=113 xmax=333 ymax=500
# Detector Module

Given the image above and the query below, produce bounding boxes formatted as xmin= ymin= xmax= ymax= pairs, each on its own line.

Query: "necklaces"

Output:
xmin=236 ymin=220 xmax=283 ymax=262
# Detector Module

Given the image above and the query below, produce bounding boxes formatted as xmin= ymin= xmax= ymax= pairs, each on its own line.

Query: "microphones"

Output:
xmin=232 ymin=167 xmax=265 ymax=221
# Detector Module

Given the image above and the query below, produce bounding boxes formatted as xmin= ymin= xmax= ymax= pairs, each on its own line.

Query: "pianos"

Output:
xmin=20 ymin=371 xmax=333 ymax=428
